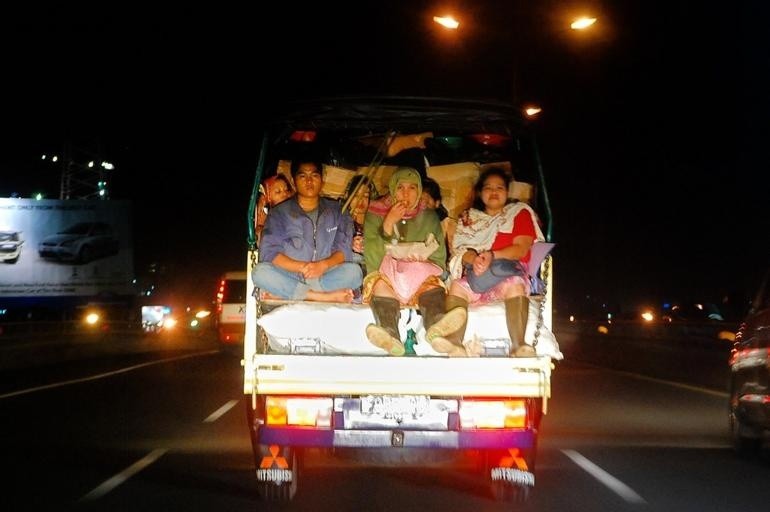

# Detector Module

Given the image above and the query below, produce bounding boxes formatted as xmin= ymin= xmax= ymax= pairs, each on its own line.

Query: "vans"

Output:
xmin=216 ymin=270 xmax=244 ymax=355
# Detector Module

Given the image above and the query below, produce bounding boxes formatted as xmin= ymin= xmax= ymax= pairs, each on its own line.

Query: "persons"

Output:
xmin=253 ymin=170 xmax=296 ymax=248
xmin=362 ymin=163 xmax=468 ymax=358
xmin=431 ymin=167 xmax=546 ymax=358
xmin=250 ymin=152 xmax=364 ymax=303
xmin=422 ymin=176 xmax=458 ymax=264
xmin=337 ymin=172 xmax=382 ymax=299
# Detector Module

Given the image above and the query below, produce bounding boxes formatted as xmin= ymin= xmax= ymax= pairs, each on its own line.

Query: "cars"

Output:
xmin=727 ymin=271 xmax=770 ymax=451
xmin=40 ymin=219 xmax=120 ymax=265
xmin=0 ymin=230 xmax=24 ymax=265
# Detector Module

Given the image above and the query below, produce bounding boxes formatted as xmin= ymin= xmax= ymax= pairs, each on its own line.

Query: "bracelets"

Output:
xmin=489 ymin=249 xmax=496 ymax=263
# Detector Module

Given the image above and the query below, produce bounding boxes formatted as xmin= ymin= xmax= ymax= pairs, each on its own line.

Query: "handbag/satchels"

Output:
xmin=377 ymin=251 xmax=442 ymax=304
xmin=463 ymin=249 xmax=525 ymax=295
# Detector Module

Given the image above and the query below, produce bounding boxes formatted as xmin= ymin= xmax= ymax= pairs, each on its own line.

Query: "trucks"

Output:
xmin=245 ymin=99 xmax=562 ymax=510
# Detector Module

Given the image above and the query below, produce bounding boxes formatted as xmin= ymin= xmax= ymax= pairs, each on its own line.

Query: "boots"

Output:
xmin=366 ymin=297 xmax=407 ymax=354
xmin=428 ymin=296 xmax=470 ymax=356
xmin=417 ymin=288 xmax=468 ymax=340
xmin=506 ymin=296 xmax=538 ymax=357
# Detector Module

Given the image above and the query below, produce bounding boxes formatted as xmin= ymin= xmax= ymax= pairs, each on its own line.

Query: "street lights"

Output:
xmin=88 ymin=161 xmax=114 ymax=198
xmin=429 ymin=6 xmax=599 ymax=102
